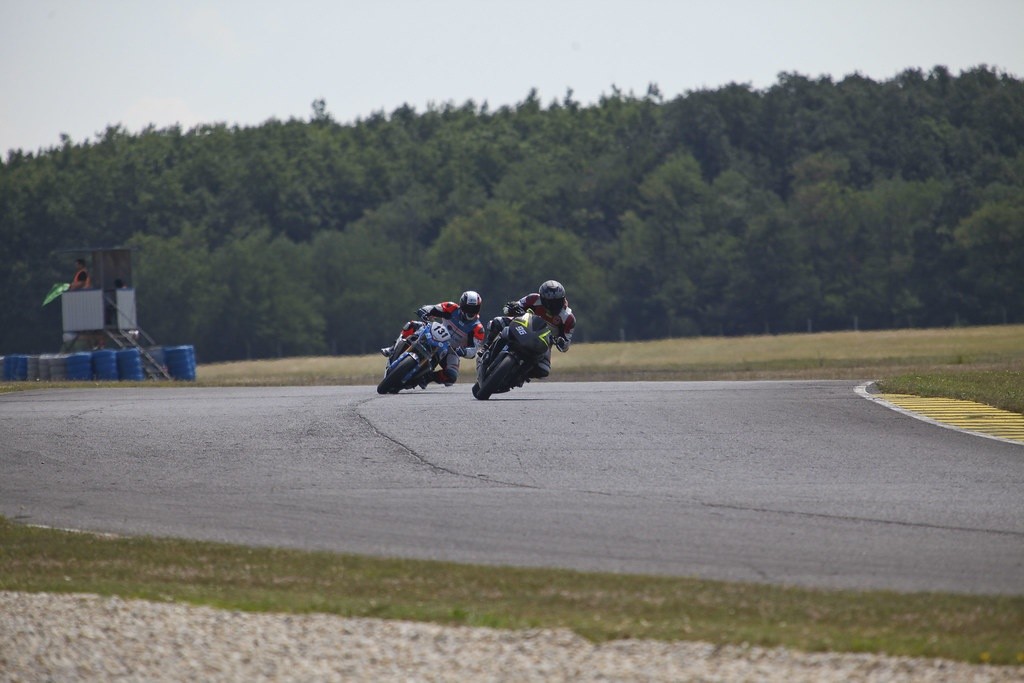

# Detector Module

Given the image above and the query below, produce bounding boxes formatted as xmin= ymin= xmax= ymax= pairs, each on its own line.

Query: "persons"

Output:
xmin=379 ymin=289 xmax=485 ymax=388
xmin=476 ymin=279 xmax=577 ymax=384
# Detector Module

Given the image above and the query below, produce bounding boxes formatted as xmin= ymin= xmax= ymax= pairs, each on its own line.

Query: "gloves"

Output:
xmin=557 ymin=337 xmax=566 ymax=347
xmin=508 ymin=300 xmax=518 ymax=311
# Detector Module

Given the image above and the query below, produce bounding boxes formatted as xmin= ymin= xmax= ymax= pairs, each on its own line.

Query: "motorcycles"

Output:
xmin=375 ymin=309 xmax=466 ymax=395
xmin=472 ymin=316 xmax=565 ymax=402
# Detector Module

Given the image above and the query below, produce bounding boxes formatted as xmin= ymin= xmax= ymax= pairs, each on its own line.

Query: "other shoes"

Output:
xmin=477 ymin=342 xmax=489 ymax=357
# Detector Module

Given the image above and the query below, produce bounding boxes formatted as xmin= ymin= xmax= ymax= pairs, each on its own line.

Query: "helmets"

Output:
xmin=539 ymin=279 xmax=566 ymax=314
xmin=460 ymin=291 xmax=482 ymax=320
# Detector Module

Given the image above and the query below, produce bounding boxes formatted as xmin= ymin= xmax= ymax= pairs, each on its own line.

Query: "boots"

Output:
xmin=420 ymin=370 xmax=437 ymax=390
xmin=380 ymin=332 xmax=404 ymax=357
